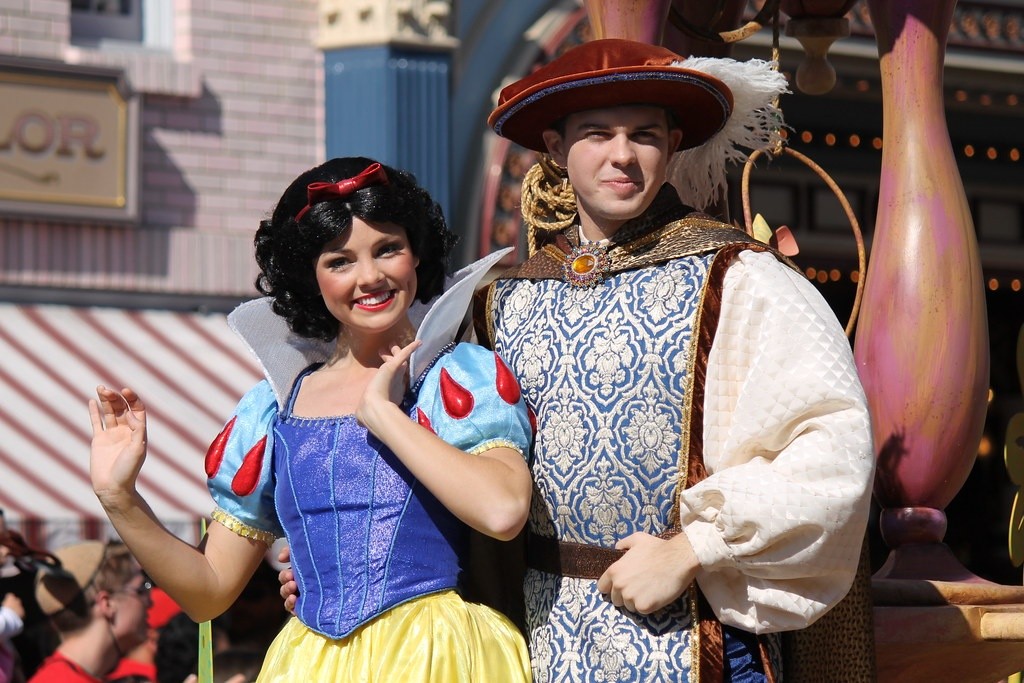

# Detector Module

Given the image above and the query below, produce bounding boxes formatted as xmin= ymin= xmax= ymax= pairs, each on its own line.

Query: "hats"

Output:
xmin=486 ymin=39 xmax=795 ymax=210
xmin=34 ymin=543 xmax=106 ymax=616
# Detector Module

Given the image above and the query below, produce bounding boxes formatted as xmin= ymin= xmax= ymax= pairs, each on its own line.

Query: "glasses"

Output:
xmin=114 ymin=579 xmax=155 ymax=594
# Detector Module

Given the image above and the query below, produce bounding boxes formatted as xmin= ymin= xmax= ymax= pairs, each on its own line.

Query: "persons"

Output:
xmin=277 ymin=39 xmax=877 ymax=683
xmin=89 ymin=159 xmax=535 ymax=683
xmin=0 ymin=508 xmax=154 ymax=683
